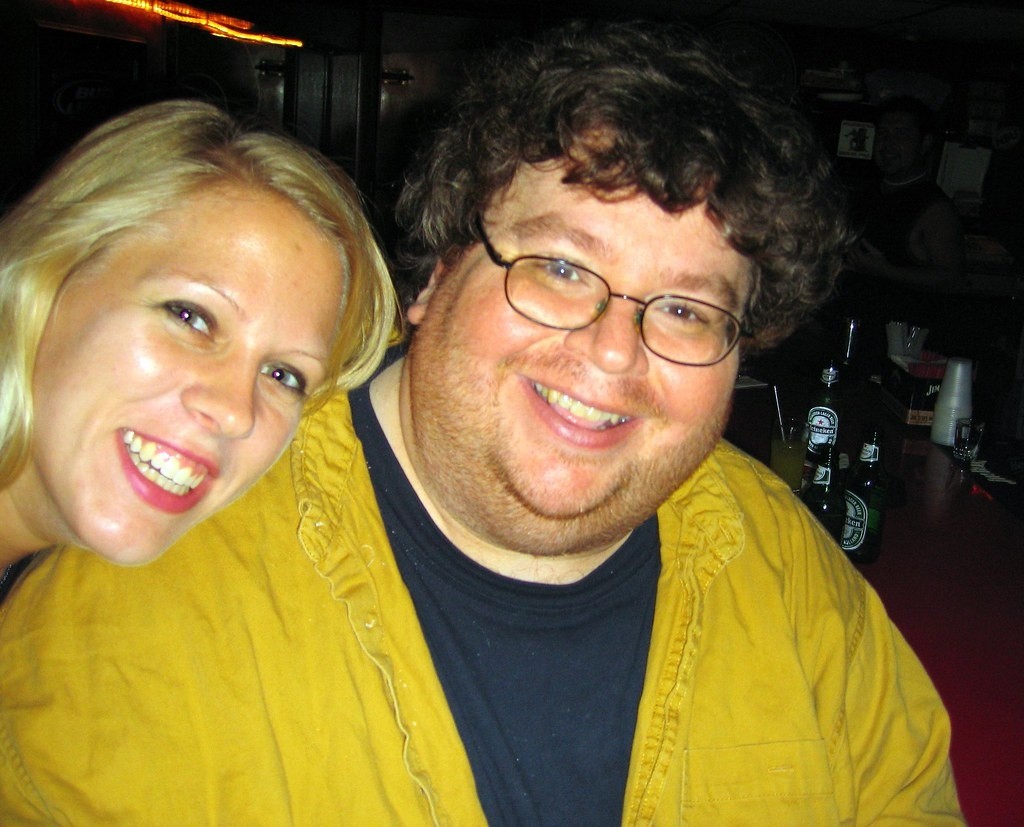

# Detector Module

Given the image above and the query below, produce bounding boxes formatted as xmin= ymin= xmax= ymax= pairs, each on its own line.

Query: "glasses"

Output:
xmin=471 ymin=210 xmax=757 ymax=367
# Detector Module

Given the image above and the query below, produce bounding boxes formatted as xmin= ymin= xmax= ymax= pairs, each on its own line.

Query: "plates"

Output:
xmin=733 ymin=375 xmax=769 ymax=389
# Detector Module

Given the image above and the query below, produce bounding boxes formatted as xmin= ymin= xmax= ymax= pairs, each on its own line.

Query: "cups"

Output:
xmin=770 ymin=417 xmax=809 ymax=496
xmin=953 ymin=418 xmax=986 ymax=460
xmin=928 ymin=357 xmax=973 ymax=449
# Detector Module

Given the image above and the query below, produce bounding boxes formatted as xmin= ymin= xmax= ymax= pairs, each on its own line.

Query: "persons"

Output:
xmin=753 ymin=94 xmax=967 ymax=357
xmin=0 ymin=40 xmax=969 ymax=826
xmin=0 ymin=100 xmax=403 ymax=569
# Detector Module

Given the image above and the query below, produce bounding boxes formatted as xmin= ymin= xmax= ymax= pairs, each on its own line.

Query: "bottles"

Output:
xmin=801 ymin=356 xmax=842 ymax=463
xmin=841 ymin=428 xmax=884 ymax=564
xmin=800 ymin=444 xmax=845 ymax=547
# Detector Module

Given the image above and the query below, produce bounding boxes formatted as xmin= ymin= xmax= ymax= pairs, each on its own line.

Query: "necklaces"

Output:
xmin=877 ymin=168 xmax=939 ymax=186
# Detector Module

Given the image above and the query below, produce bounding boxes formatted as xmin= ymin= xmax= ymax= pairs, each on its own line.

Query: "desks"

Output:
xmin=722 ymin=350 xmax=1024 ymax=827
